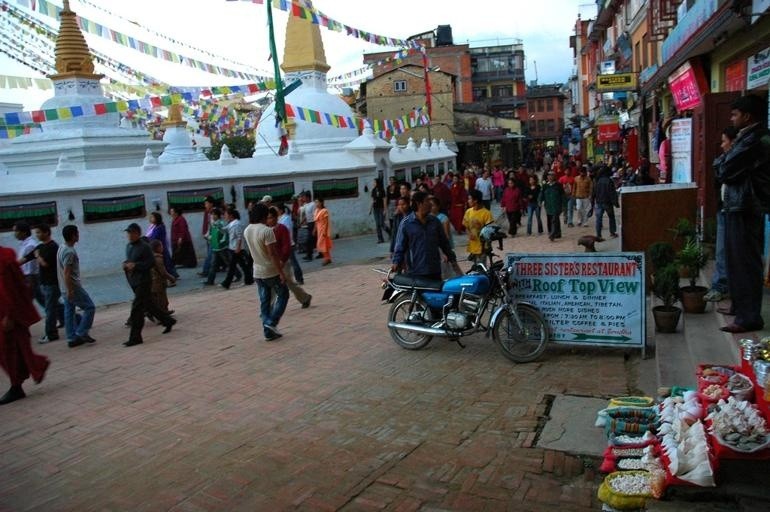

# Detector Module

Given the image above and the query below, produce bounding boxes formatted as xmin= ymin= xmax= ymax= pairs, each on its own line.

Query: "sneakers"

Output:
xmin=262 ymin=322 xmax=282 ymax=342
xmin=701 ymin=283 xmax=730 ymax=302
xmin=168 ymin=308 xmax=177 ymax=316
xmin=146 ymin=313 xmax=156 ymax=323
xmin=194 ymin=265 xmax=255 ymax=290
xmin=302 ymin=294 xmax=312 ymax=308
xmin=298 ymin=247 xmax=332 ymax=267
xmin=37 ymin=361 xmax=50 ymax=385
xmin=0 ymin=388 xmax=27 ymax=405
xmin=509 ymin=219 xmax=619 ymax=242
xmin=37 ymin=334 xmax=60 ymax=344
xmin=55 ymin=321 xmax=66 ymax=328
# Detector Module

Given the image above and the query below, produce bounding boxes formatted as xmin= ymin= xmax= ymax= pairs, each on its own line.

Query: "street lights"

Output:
xmin=424 ymin=65 xmax=441 ymax=147
xmin=527 ymin=112 xmax=535 ymax=135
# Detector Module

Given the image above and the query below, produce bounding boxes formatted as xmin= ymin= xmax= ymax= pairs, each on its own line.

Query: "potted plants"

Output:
xmin=645 ymin=242 xmax=681 ymax=333
xmin=666 ymin=218 xmax=708 ymax=313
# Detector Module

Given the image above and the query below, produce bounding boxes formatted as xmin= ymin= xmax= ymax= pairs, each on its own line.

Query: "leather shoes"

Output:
xmin=716 ymin=306 xmax=731 ymax=314
xmin=76 ymin=332 xmax=96 ymax=344
xmin=121 ymin=338 xmax=144 ymax=347
xmin=718 ymin=318 xmax=765 ymax=334
xmin=67 ymin=336 xmax=86 ymax=348
xmin=161 ymin=319 xmax=178 ymax=334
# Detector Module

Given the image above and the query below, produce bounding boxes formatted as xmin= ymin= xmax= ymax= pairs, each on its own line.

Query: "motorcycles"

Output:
xmin=372 ymin=221 xmax=549 ymax=364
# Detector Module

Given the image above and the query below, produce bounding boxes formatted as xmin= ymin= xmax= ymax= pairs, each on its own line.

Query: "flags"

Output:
xmin=0 ymin=0 xmax=432 ymax=143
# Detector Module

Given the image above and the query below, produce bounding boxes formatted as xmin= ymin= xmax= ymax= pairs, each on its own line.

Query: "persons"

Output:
xmin=122 ymin=206 xmax=198 ymax=346
xmin=0 ymin=223 xmax=96 ymax=402
xmin=702 ymin=130 xmax=733 ymax=300
xmin=196 ymin=190 xmax=332 ymax=340
xmin=713 ymin=97 xmax=764 ymax=333
xmin=367 ymin=154 xmax=619 ymax=340
